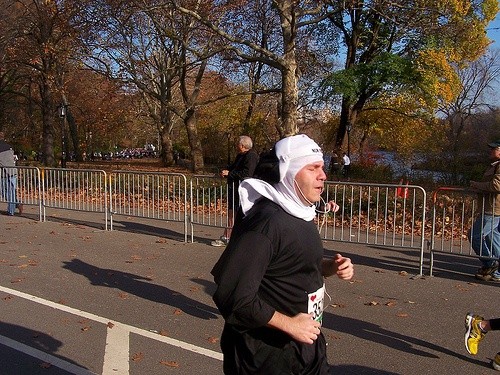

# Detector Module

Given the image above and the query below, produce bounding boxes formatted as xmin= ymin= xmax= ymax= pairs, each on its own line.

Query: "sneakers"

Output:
xmin=477 ymin=260 xmax=500 ymax=281
xmin=464 ymin=313 xmax=486 ymax=355
xmin=211 ymin=236 xmax=230 ymax=246
xmin=492 ymin=353 xmax=500 ymax=370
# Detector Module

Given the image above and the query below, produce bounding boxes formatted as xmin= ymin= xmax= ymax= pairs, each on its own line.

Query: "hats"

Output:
xmin=487 ymin=140 xmax=500 ymax=147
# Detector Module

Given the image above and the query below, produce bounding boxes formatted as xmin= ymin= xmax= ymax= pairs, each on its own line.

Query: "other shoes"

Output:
xmin=8 ymin=213 xmax=14 ymax=216
xmin=19 ymin=204 xmax=23 ymax=215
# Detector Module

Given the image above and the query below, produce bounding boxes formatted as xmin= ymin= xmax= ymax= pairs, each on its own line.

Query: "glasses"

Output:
xmin=488 ymin=147 xmax=500 ymax=150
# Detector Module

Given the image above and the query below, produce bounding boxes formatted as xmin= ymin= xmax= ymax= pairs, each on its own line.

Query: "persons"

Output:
xmin=470 ymin=140 xmax=500 ymax=283
xmin=0 ymin=139 xmax=23 ymax=216
xmin=329 ymin=152 xmax=351 ymax=180
xmin=211 ymin=134 xmax=354 ymax=375
xmin=464 ymin=312 xmax=500 ymax=371
xmin=210 ymin=135 xmax=256 ymax=247
